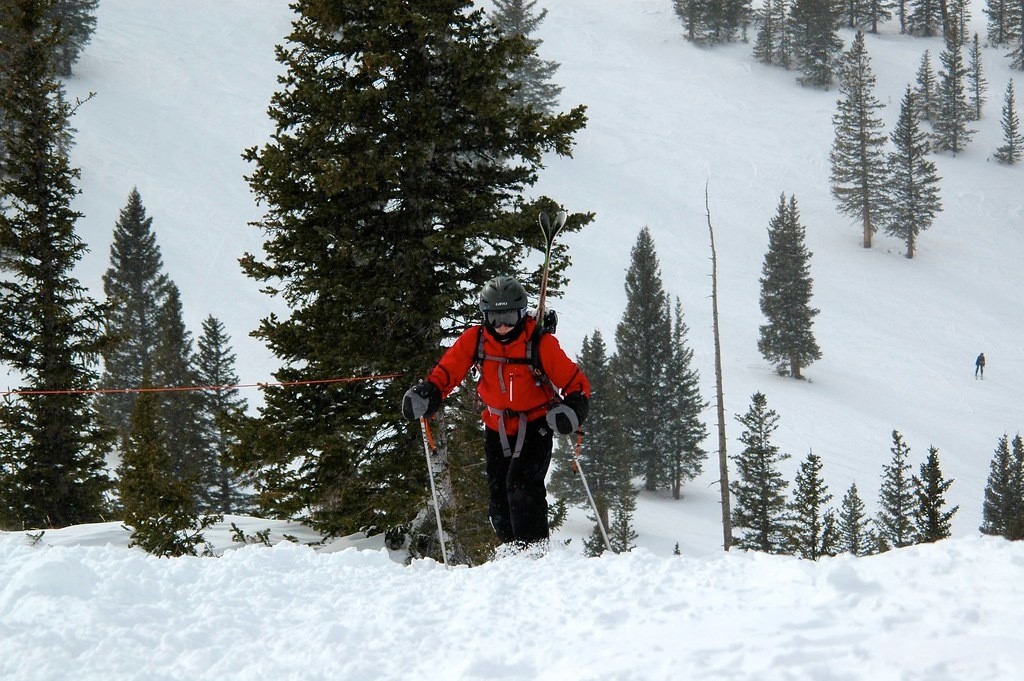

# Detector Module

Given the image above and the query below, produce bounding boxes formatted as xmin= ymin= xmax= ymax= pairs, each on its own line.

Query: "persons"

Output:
xmin=401 ymin=275 xmax=591 ymax=546
xmin=975 ymin=353 xmax=985 ymax=380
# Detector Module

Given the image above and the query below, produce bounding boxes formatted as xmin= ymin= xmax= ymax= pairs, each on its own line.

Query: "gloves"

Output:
xmin=546 ymin=400 xmax=586 ymax=434
xmin=402 ymin=381 xmax=442 ymax=420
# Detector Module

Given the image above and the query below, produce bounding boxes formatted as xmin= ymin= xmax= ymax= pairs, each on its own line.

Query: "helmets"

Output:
xmin=479 ymin=275 xmax=528 ymax=310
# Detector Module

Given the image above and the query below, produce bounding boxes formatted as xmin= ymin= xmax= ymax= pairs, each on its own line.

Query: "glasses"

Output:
xmin=488 ymin=309 xmax=518 ymax=327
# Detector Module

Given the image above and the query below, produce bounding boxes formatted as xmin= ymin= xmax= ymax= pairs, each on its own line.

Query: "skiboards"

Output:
xmin=535 ymin=210 xmax=567 ymax=334
